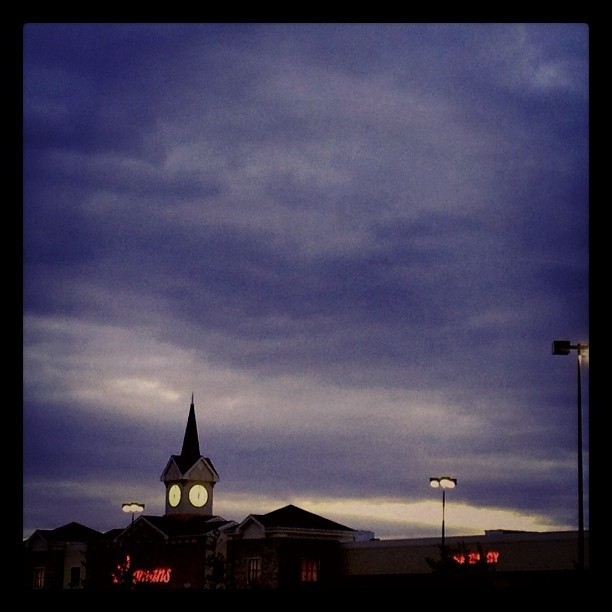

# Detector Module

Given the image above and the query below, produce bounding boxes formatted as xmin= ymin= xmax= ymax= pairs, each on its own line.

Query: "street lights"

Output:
xmin=121 ymin=501 xmax=145 ymax=523
xmin=429 ymin=476 xmax=457 ymax=543
xmin=551 ymin=339 xmax=591 ymax=569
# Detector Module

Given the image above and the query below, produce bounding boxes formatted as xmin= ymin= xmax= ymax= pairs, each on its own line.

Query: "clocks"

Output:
xmin=168 ymin=484 xmax=181 ymax=507
xmin=189 ymin=485 xmax=209 ymax=508
